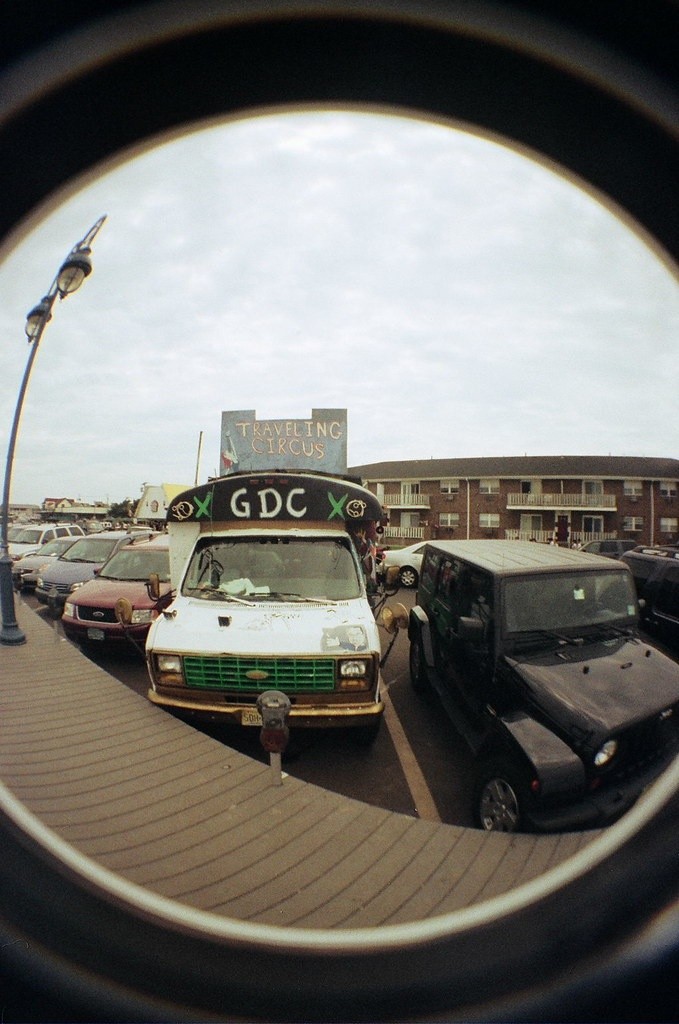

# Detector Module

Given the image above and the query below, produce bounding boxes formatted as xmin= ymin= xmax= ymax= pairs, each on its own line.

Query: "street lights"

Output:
xmin=0 ymin=212 xmax=110 ymax=649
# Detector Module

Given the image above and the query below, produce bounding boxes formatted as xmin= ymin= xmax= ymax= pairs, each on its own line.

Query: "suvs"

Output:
xmin=34 ymin=526 xmax=167 ymax=609
xmin=407 ymin=538 xmax=679 ymax=835
xmin=61 ymin=531 xmax=171 ymax=651
xmin=579 ymin=538 xmax=679 ymax=643
xmin=6 ymin=522 xmax=86 ymax=566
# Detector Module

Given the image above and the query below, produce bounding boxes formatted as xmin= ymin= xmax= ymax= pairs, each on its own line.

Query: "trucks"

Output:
xmin=144 ymin=409 xmax=409 ymax=731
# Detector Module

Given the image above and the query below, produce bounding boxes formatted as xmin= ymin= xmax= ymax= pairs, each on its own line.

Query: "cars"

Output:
xmin=382 ymin=540 xmax=426 ymax=591
xmin=7 ymin=513 xmax=168 ymax=537
xmin=12 ymin=535 xmax=91 ymax=592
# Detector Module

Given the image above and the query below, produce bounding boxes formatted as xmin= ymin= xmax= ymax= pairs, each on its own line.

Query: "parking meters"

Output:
xmin=255 ymin=688 xmax=290 ymax=788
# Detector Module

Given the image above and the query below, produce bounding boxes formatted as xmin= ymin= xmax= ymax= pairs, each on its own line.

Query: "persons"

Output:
xmin=150 ymin=521 xmax=168 ymax=534
xmin=572 ymin=539 xmax=581 ymax=550
xmin=547 ymin=537 xmax=558 ymax=547
